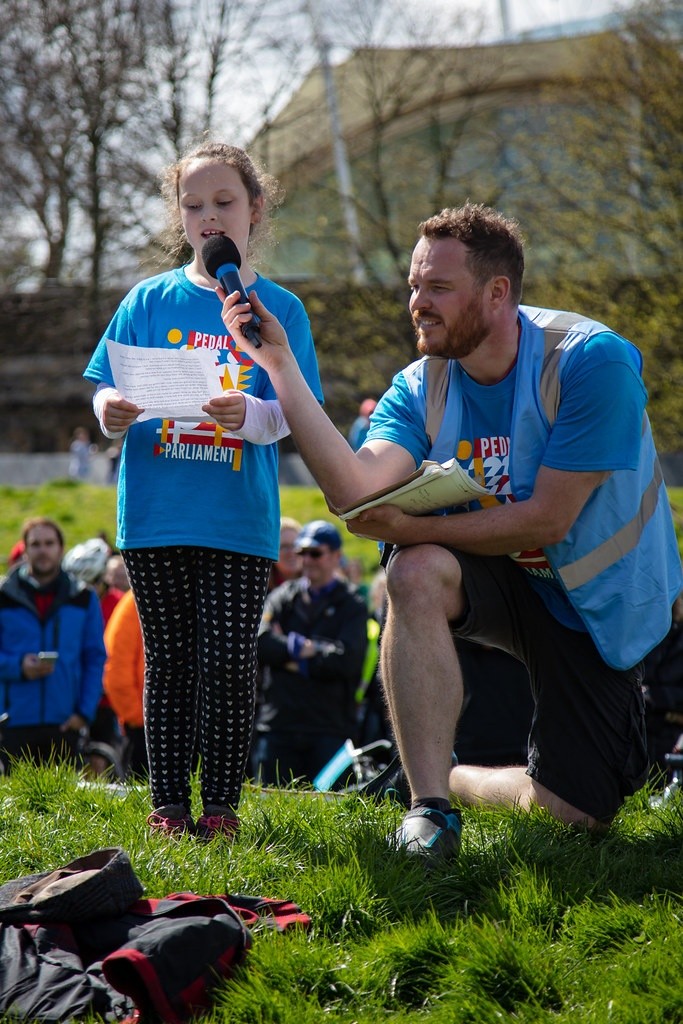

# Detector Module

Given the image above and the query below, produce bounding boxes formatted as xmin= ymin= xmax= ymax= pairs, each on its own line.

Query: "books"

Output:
xmin=338 ymin=458 xmax=489 ymax=520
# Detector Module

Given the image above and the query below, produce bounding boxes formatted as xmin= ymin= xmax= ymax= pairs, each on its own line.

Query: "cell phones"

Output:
xmin=37 ymin=652 xmax=58 ymax=665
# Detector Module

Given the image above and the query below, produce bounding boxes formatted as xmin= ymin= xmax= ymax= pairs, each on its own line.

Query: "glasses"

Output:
xmin=296 ymin=547 xmax=334 ymax=558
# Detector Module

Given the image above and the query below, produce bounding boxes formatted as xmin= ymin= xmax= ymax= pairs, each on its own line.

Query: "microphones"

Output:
xmin=201 ymin=235 xmax=262 ymax=349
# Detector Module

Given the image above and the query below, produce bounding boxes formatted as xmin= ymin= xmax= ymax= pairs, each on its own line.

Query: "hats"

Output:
xmin=294 ymin=520 xmax=344 ymax=549
xmin=61 ymin=537 xmax=110 ymax=592
xmin=362 ymin=399 xmax=381 ymax=418
xmin=9 ymin=539 xmax=25 ymax=566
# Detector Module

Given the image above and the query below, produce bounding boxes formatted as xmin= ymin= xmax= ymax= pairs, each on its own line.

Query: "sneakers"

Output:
xmin=196 ymin=806 xmax=243 ymax=848
xmin=147 ymin=805 xmax=196 ymax=848
xmin=389 ymin=805 xmax=463 ymax=865
xmin=340 ymin=746 xmax=459 ymax=815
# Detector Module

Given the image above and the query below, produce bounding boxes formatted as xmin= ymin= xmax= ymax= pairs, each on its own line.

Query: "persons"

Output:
xmin=106 ymin=440 xmax=123 ymax=484
xmin=0 ymin=518 xmax=149 ymax=783
xmin=349 ymin=399 xmax=376 ymax=453
xmin=70 ymin=433 xmax=91 ymax=477
xmin=245 ymin=518 xmax=391 ymax=790
xmin=215 ymin=204 xmax=683 ymax=855
xmin=84 ymin=143 xmax=324 ymax=843
xmin=643 ymin=596 xmax=683 ymax=790
